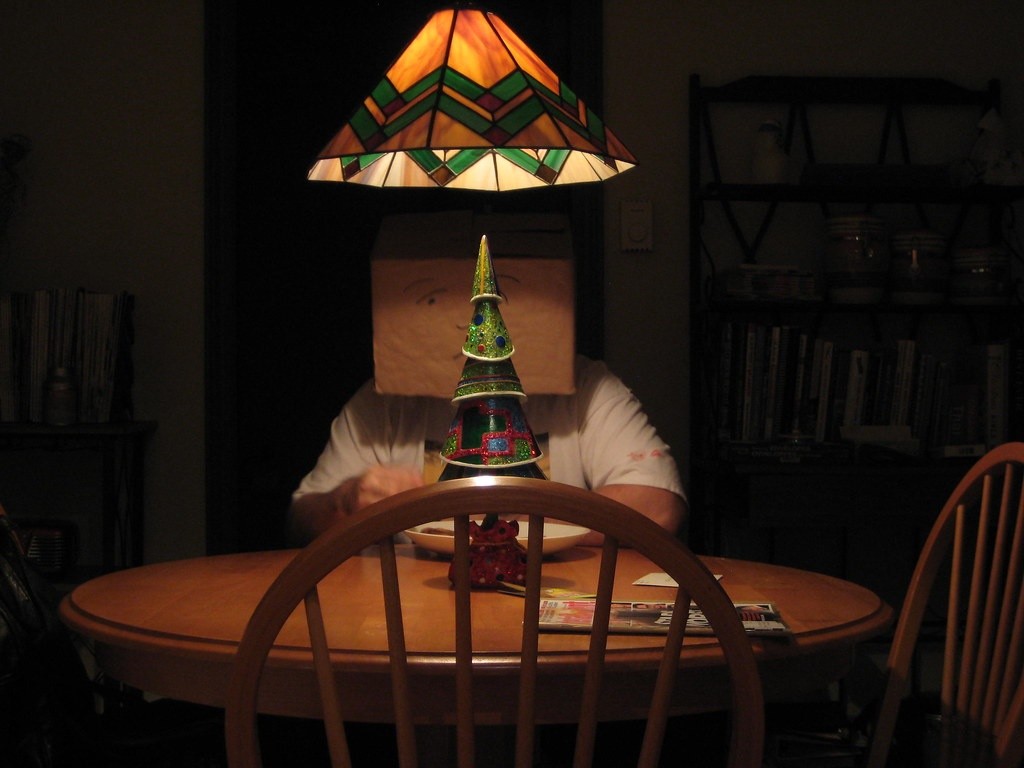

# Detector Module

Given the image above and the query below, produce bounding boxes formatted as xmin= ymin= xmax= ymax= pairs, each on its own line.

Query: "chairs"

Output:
xmin=689 ymin=442 xmax=1024 ymax=768
xmin=224 ymin=473 xmax=766 ymax=768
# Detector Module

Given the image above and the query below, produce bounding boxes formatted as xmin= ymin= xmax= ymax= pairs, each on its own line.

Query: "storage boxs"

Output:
xmin=373 ymin=214 xmax=581 ymax=396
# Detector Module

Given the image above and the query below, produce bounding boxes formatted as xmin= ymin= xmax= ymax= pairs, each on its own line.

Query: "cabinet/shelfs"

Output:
xmin=688 ymin=70 xmax=1024 ymax=691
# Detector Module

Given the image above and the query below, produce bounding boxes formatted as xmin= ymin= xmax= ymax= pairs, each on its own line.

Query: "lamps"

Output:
xmin=306 ymin=0 xmax=640 ymax=193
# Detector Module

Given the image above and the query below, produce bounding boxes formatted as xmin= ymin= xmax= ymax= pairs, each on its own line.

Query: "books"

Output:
xmin=706 ymin=321 xmax=1008 ymax=464
xmin=537 ymin=591 xmax=793 ymax=638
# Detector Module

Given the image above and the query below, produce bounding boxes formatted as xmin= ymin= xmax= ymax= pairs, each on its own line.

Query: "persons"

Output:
xmin=286 ymin=211 xmax=692 ymax=546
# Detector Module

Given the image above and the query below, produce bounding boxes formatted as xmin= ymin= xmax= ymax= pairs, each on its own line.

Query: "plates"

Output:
xmin=403 ymin=517 xmax=592 ymax=558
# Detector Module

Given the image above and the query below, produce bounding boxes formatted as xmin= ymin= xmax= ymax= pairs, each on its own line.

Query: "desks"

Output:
xmin=0 ymin=420 xmax=162 ymax=755
xmin=59 ymin=546 xmax=895 ymax=768
